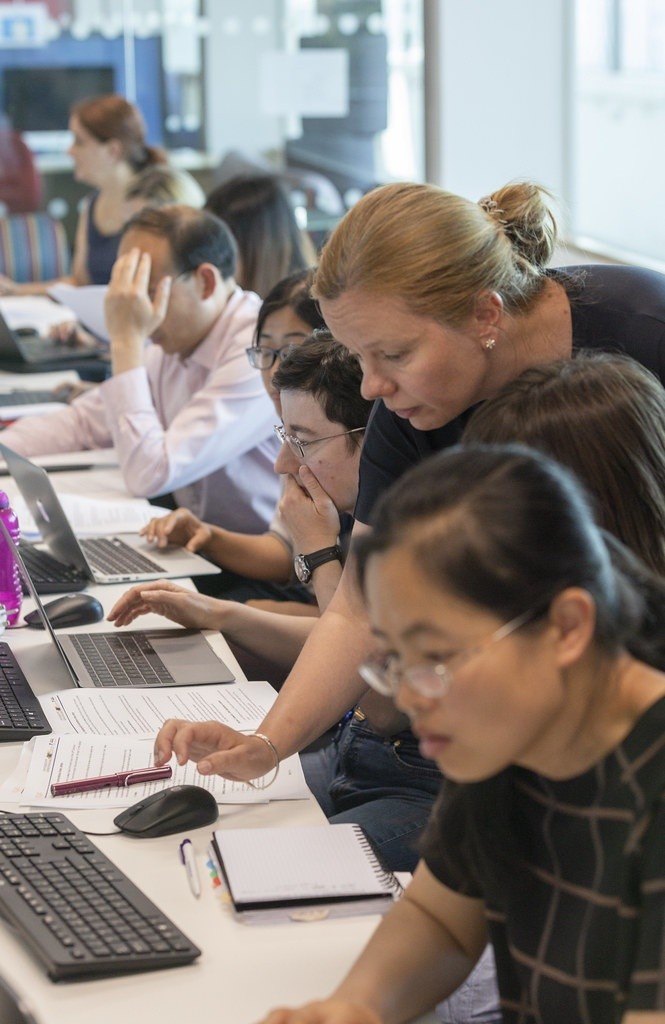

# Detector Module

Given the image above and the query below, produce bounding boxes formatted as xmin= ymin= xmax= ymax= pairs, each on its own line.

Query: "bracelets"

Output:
xmin=247 ymin=735 xmax=279 ymax=788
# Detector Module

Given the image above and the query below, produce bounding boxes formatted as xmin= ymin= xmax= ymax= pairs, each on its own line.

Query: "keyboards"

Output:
xmin=1 ymin=815 xmax=203 ymax=981
xmin=13 ymin=538 xmax=85 ymax=594
xmin=0 ymin=642 xmax=53 ymax=742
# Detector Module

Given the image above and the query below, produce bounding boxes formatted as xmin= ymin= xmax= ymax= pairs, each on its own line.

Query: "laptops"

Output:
xmin=0 ymin=520 xmax=234 ymax=689
xmin=0 ymin=314 xmax=221 ymax=584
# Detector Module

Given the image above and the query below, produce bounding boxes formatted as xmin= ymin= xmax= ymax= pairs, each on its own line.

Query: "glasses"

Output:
xmin=246 ymin=345 xmax=297 ymax=370
xmin=273 ymin=423 xmax=368 ymax=461
xmin=356 ymin=580 xmax=578 ymax=697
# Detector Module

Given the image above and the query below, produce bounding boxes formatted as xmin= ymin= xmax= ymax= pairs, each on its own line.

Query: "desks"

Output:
xmin=0 ymin=373 xmax=378 ymax=1024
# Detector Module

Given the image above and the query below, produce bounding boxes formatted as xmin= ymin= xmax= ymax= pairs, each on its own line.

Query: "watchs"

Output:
xmin=294 ymin=545 xmax=342 ymax=584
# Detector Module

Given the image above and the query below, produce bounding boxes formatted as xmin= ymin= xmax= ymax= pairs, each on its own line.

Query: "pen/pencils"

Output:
xmin=180 ymin=839 xmax=200 ymax=899
xmin=51 ymin=765 xmax=172 ymax=797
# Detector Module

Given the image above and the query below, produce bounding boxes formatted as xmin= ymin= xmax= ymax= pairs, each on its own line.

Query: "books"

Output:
xmin=211 ymin=823 xmax=397 ymax=925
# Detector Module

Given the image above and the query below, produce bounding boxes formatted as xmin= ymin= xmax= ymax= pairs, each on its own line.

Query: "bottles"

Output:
xmin=0 ymin=490 xmax=23 ymax=625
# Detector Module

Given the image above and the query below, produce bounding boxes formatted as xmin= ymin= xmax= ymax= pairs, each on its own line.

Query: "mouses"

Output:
xmin=14 ymin=328 xmax=38 ymax=336
xmin=24 ymin=593 xmax=103 ymax=629
xmin=112 ymin=784 xmax=219 ymax=838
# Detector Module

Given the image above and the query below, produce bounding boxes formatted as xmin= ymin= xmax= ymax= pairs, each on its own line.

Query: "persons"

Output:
xmin=0 ymin=207 xmax=286 ymax=535
xmin=105 ymin=330 xmax=445 ymax=869
xmin=0 ymin=95 xmax=166 ymax=297
xmin=138 ymin=265 xmax=333 ymax=616
xmin=442 ymin=353 xmax=665 ymax=1023
xmin=201 ymin=174 xmax=303 ymax=298
xmin=252 ymin=447 xmax=665 ymax=1024
xmin=151 ymin=184 xmax=664 ymax=781
xmin=128 ymin=164 xmax=204 ymax=212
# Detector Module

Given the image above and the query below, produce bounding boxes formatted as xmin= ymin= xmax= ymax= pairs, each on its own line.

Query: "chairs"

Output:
xmin=204 ymin=165 xmax=279 ymax=250
